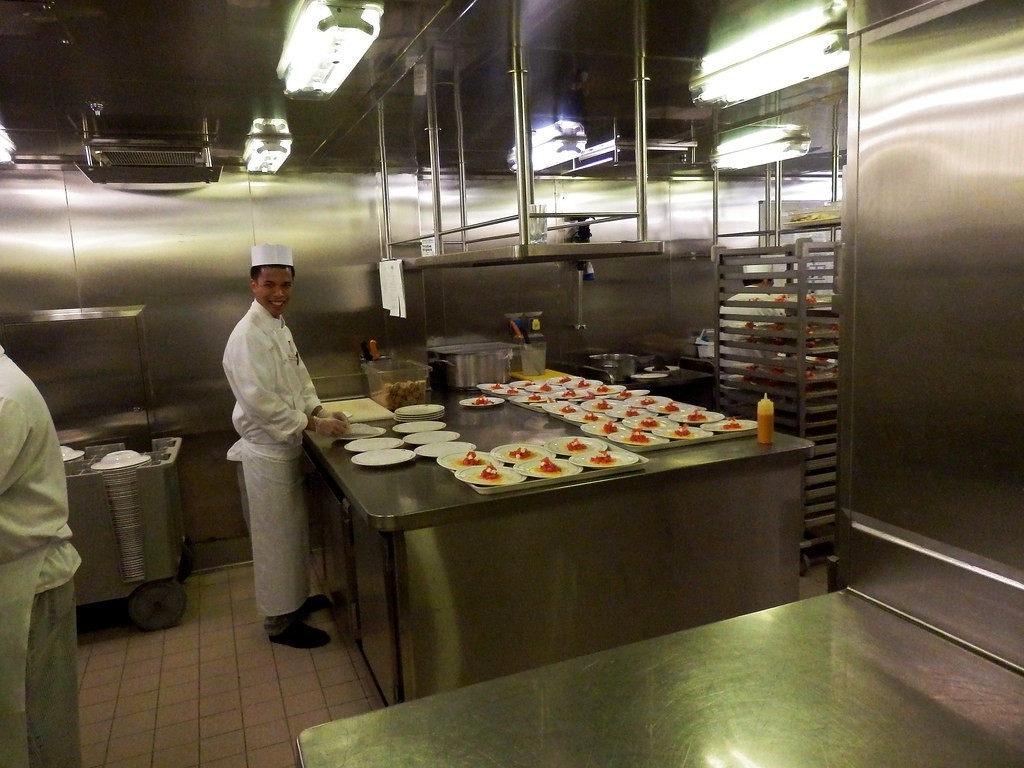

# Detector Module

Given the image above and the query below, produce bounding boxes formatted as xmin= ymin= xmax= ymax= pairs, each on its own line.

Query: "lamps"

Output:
xmin=243 ymin=140 xmax=292 ymax=174
xmin=249 ymin=118 xmax=292 ymax=138
xmin=507 ymin=137 xmax=588 ymax=173
xmin=506 ymin=120 xmax=587 ymax=162
xmin=709 ymin=138 xmax=811 ymax=170
xmin=709 ymin=124 xmax=810 ymax=158
xmin=691 ymin=0 xmax=848 ymax=82
xmin=284 ymin=6 xmax=382 ymax=102
xmin=688 ymin=27 xmax=850 ymax=108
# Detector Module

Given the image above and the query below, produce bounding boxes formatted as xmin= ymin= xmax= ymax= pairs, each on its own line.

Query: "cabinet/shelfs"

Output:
xmin=300 ymin=377 xmax=815 ymax=707
xmin=709 ymin=237 xmax=840 ymax=576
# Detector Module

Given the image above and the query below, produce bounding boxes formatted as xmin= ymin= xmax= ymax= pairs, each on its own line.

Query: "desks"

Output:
xmin=297 ymin=590 xmax=1024 ymax=768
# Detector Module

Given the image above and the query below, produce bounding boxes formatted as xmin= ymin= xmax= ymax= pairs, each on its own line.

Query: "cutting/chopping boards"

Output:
xmin=322 ymin=397 xmax=395 ymax=422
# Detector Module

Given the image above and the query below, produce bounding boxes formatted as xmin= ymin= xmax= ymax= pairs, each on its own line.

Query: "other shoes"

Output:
xmin=290 ymin=594 xmax=329 ymax=620
xmin=268 ymin=620 xmax=331 ymax=648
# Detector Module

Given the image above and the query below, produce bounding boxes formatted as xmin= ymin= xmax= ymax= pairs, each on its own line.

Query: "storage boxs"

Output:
xmin=362 ymin=359 xmax=434 ymax=412
xmin=428 ymin=341 xmax=523 ymax=391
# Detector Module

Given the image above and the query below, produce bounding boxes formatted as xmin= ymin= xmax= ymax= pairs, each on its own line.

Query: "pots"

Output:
xmin=589 ymin=352 xmax=636 ymax=382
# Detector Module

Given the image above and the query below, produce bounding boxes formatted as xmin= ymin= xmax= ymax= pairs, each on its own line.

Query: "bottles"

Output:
xmin=757 ymin=392 xmax=774 ymax=445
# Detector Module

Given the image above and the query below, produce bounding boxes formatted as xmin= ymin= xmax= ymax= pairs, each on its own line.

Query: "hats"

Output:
xmin=743 ymin=263 xmax=774 ymax=273
xmin=251 ymin=243 xmax=293 ymax=266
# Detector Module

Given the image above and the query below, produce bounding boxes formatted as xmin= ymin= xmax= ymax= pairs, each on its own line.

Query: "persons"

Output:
xmin=720 ymin=265 xmax=785 ymax=378
xmin=0 ymin=345 xmax=82 ymax=768
xmin=222 ymin=242 xmax=350 ymax=649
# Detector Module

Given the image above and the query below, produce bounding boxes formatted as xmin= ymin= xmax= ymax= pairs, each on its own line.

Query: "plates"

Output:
xmin=340 ymin=427 xmax=387 ymax=441
xmin=58 ymin=445 xmax=86 ymax=476
xmin=90 ymin=449 xmax=155 ymax=578
xmin=351 ymin=449 xmax=417 ymax=467
xmin=630 ymin=366 xmax=680 ymax=378
xmin=344 ymin=437 xmax=403 ymax=453
xmin=393 ymin=373 xmax=757 ymax=487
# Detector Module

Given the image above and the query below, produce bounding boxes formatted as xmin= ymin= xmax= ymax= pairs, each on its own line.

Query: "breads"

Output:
xmin=382 ymin=380 xmax=425 ymax=411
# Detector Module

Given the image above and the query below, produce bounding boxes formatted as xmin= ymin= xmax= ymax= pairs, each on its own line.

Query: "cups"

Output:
xmin=529 ymin=203 xmax=548 ymax=243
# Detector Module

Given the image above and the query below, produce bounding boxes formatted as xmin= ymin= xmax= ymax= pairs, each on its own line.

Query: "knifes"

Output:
xmin=359 ymin=339 xmax=380 ymax=364
